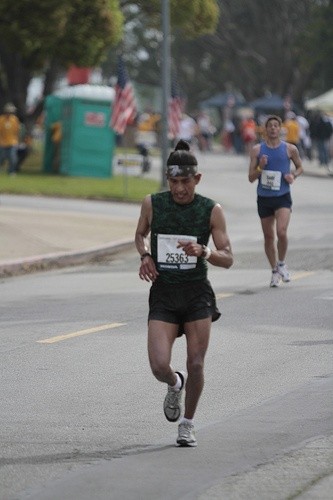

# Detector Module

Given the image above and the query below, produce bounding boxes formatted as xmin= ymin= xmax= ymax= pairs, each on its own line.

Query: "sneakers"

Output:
xmin=162 ymin=371 xmax=186 ymax=422
xmin=175 ymin=422 xmax=198 ymax=447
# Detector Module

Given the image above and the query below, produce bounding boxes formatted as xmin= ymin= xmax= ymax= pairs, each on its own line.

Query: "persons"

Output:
xmin=134 ymin=141 xmax=233 ymax=444
xmin=0 ymin=104 xmax=333 ymax=175
xmin=248 ymin=116 xmax=303 ymax=287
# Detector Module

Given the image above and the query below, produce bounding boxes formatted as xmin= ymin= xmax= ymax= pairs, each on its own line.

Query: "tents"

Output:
xmin=200 ymin=88 xmax=333 ymax=108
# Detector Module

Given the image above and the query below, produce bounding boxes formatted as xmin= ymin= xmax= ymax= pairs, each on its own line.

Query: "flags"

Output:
xmin=167 ymin=77 xmax=182 ymax=139
xmin=108 ymin=60 xmax=138 ymax=134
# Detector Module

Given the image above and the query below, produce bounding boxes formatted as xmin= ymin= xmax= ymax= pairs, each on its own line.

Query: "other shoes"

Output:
xmin=270 ymin=273 xmax=280 ymax=287
xmin=278 ymin=265 xmax=290 ymax=282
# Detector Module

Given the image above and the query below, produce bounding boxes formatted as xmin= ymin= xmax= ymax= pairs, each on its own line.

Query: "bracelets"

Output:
xmin=257 ymin=166 xmax=263 ymax=172
xmin=202 ymin=245 xmax=212 ymax=259
xmin=293 ymin=172 xmax=297 ymax=179
xmin=141 ymin=254 xmax=150 ymax=260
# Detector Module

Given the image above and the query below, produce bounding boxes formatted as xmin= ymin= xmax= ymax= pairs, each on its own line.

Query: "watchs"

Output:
xmin=201 ymin=243 xmax=206 ymax=257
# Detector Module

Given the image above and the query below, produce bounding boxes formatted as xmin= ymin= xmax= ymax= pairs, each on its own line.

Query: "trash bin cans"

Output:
xmin=43 ymin=84 xmax=117 ymax=179
